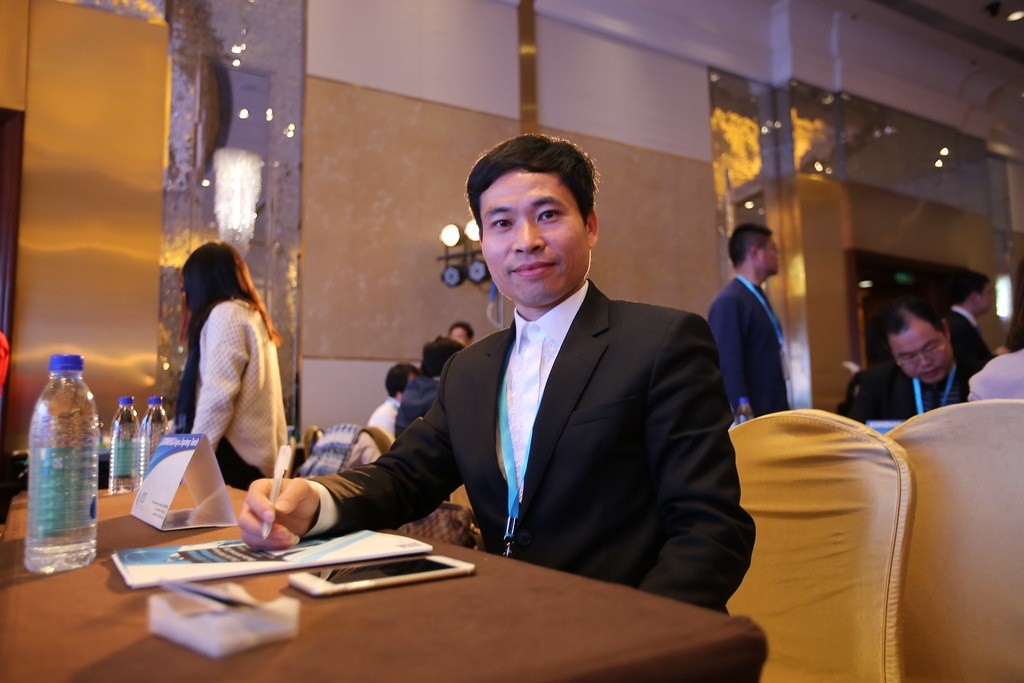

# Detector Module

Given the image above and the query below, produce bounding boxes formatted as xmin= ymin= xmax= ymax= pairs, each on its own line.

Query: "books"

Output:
xmin=111 ymin=529 xmax=433 ymax=589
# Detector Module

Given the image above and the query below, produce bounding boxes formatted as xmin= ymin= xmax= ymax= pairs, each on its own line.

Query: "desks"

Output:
xmin=0 ymin=482 xmax=767 ymax=683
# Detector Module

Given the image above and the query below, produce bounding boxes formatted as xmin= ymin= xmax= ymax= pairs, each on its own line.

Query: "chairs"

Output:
xmin=303 ymin=424 xmax=325 ymax=459
xmin=723 ymin=409 xmax=917 ymax=683
xmin=884 ymin=399 xmax=1024 ymax=683
xmin=364 ymin=424 xmax=395 ymax=453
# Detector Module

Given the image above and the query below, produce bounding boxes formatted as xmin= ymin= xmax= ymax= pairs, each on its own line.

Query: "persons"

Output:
xmin=448 ymin=320 xmax=474 ymax=346
xmin=239 ymin=133 xmax=757 ymax=616
xmin=361 ymin=362 xmax=420 ymax=464
xmin=945 ymin=267 xmax=1008 ymax=375
xmin=179 ymin=241 xmax=288 ymax=493
xmin=396 ymin=336 xmax=466 ymax=438
xmin=968 ymin=257 xmax=1024 ymax=401
xmin=708 ymin=223 xmax=790 ymax=426
xmin=838 ymin=296 xmax=970 ymax=425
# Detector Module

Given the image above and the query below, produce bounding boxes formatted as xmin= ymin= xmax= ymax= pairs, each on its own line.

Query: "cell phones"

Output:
xmin=288 ymin=554 xmax=476 ymax=597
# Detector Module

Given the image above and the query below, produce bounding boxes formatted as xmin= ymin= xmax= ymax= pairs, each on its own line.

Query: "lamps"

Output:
xmin=439 ymin=224 xmax=466 ymax=248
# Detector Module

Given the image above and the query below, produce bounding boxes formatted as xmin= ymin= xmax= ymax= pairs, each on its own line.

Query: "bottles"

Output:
xmin=24 ymin=354 xmax=100 ymax=574
xmin=141 ymin=397 xmax=168 ymax=485
xmin=735 ymin=398 xmax=752 ymax=426
xmin=110 ymin=397 xmax=139 ymax=494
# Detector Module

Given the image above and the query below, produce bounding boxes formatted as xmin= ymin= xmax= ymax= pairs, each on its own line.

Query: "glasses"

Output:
xmin=890 ymin=331 xmax=947 ymax=366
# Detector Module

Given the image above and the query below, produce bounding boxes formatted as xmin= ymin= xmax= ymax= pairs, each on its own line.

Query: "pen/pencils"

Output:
xmin=261 ymin=444 xmax=292 ymax=538
